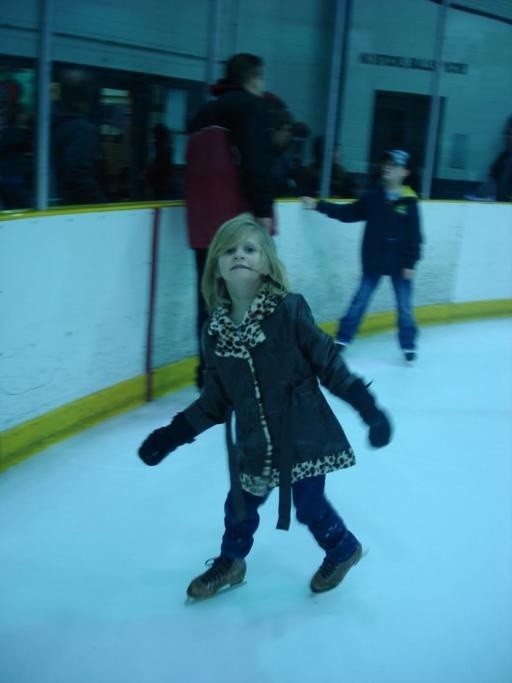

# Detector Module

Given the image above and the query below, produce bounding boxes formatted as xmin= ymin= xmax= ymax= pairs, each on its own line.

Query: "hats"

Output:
xmin=386 ymin=147 xmax=414 ymax=169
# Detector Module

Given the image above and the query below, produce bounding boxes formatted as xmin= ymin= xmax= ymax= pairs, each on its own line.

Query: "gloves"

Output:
xmin=347 ymin=378 xmax=393 ymax=448
xmin=139 ymin=411 xmax=196 ymax=467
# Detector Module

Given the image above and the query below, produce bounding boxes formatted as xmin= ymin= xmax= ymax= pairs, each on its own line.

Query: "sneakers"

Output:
xmin=187 ymin=555 xmax=246 ymax=597
xmin=311 ymin=538 xmax=361 ymax=593
xmin=334 ymin=341 xmax=346 ymax=354
xmin=405 ymin=350 xmax=416 ymax=361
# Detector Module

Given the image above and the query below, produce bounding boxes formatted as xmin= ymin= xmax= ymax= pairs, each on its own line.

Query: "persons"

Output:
xmin=264 ymin=94 xmax=356 ymax=200
xmin=189 ymin=51 xmax=279 ymax=393
xmin=133 ymin=211 xmax=388 ymax=600
xmin=2 ymin=58 xmax=188 ymax=211
xmin=484 ymin=116 xmax=512 ymax=205
xmin=298 ymin=141 xmax=426 ymax=362
